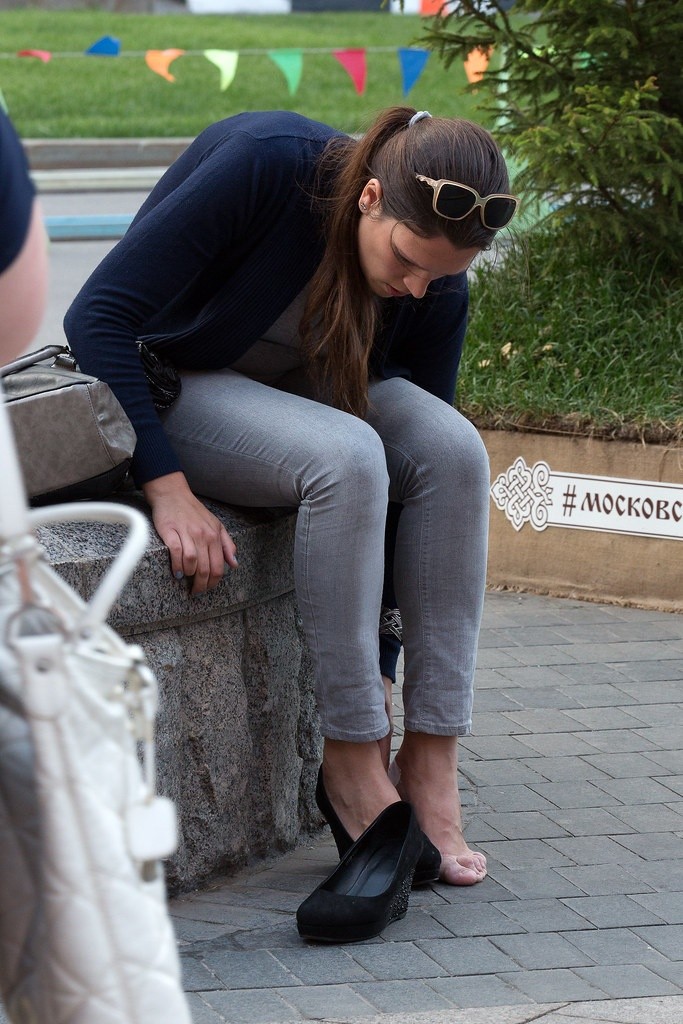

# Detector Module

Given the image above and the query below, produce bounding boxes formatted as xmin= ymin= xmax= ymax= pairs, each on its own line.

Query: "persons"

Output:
xmin=62 ymin=103 xmax=520 ymax=886
xmin=1 ymin=104 xmax=51 ymax=366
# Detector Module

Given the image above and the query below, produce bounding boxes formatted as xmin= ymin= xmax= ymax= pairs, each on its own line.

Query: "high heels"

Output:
xmin=316 ymin=764 xmax=442 ymax=886
xmin=297 ymin=799 xmax=419 ymax=941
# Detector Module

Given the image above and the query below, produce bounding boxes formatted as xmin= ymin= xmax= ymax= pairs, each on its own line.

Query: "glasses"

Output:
xmin=414 ymin=173 xmax=518 ymax=231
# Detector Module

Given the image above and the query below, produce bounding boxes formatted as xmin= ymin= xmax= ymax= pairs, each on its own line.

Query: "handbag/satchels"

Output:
xmin=0 ymin=500 xmax=189 ymax=1024
xmin=0 ymin=345 xmax=137 ymax=499
